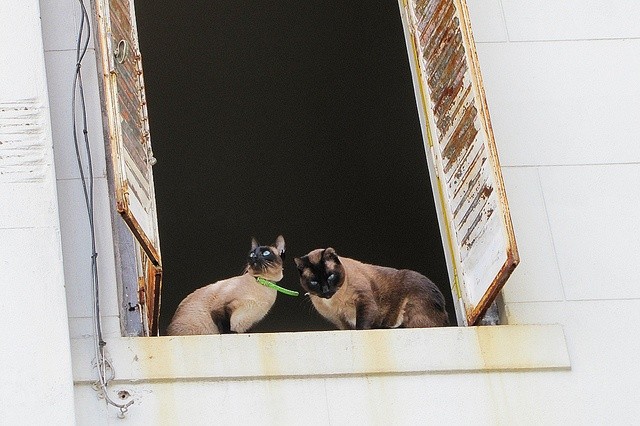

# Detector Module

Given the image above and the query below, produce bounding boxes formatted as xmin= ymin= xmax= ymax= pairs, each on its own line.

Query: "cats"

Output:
xmin=294 ymin=248 xmax=450 ymax=329
xmin=167 ymin=235 xmax=286 ymax=336
